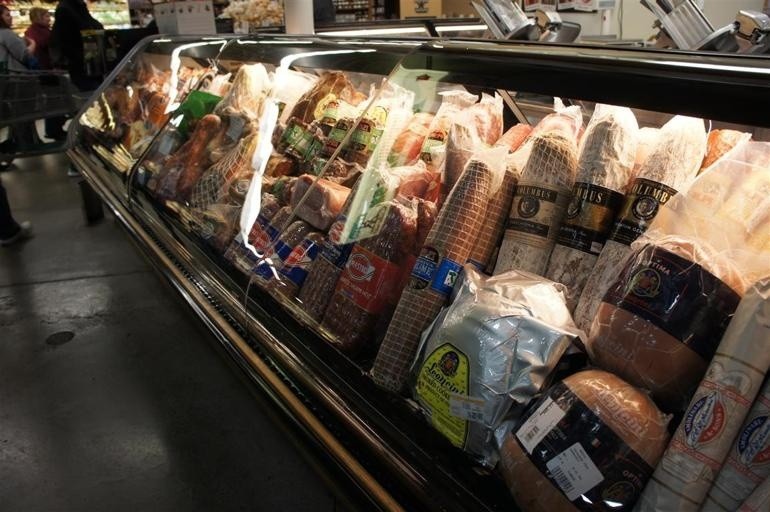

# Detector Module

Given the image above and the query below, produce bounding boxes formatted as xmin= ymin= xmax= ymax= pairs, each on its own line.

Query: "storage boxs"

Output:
xmin=154 ymin=0 xmax=219 ymax=39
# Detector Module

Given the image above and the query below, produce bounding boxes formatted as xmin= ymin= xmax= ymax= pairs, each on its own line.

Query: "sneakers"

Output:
xmin=0 ymin=220 xmax=32 ymax=245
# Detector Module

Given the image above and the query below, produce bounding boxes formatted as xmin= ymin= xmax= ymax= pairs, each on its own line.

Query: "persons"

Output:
xmin=0 ymin=1 xmax=106 ymax=246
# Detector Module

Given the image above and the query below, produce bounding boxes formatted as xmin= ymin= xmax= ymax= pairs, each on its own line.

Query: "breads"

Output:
xmin=501 ymin=369 xmax=670 ymax=510
xmin=588 ymin=237 xmax=745 ymax=394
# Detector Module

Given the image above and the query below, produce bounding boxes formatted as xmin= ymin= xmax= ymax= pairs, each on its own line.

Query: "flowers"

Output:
xmin=216 ymin=0 xmax=285 ymax=27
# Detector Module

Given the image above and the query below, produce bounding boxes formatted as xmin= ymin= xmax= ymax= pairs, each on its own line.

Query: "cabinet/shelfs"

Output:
xmin=63 ymin=23 xmax=767 ymax=511
